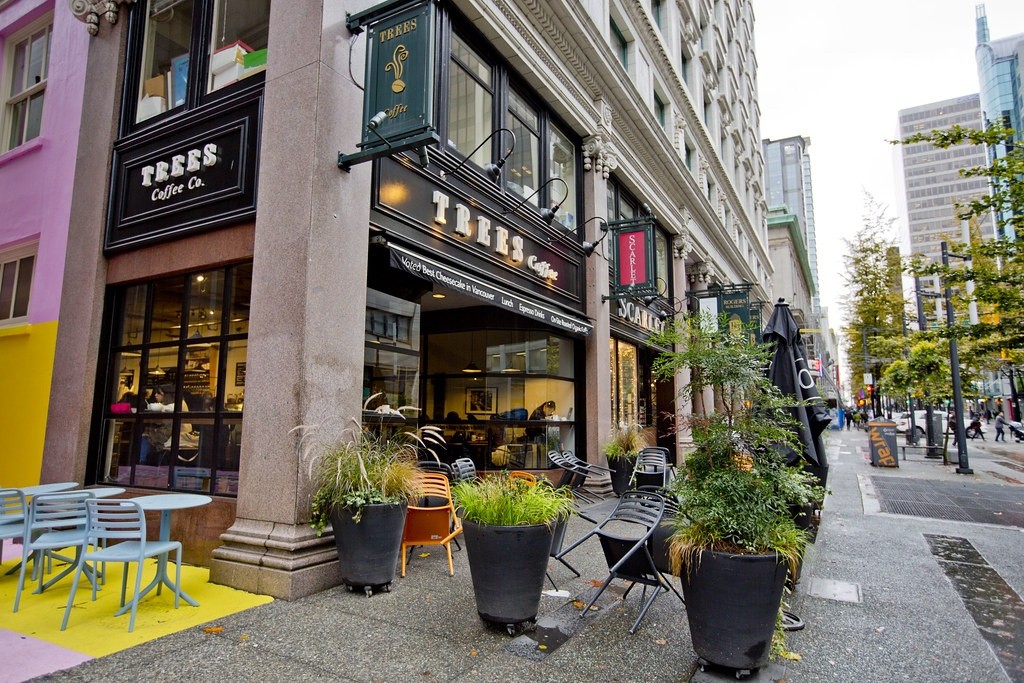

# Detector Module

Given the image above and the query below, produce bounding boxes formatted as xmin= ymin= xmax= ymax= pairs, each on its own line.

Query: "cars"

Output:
xmin=896 ymin=410 xmax=988 ymax=438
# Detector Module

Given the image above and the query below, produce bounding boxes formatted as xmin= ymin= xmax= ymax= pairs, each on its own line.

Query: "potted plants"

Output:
xmin=288 ymin=391 xmax=426 ymax=596
xmin=601 ymin=422 xmax=650 ymax=497
xmin=448 ymin=471 xmax=579 ymax=638
xmin=649 ymin=311 xmax=833 ymax=683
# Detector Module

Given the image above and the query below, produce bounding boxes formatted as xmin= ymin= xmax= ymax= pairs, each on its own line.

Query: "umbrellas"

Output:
xmin=743 ymin=297 xmax=831 ymax=544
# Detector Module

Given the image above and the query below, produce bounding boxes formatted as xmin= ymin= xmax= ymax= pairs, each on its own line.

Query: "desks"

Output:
xmin=422 ymin=421 xmax=575 ymax=468
xmin=113 ymin=494 xmax=212 ymax=616
xmin=3 ymin=481 xmax=78 ymax=576
xmin=32 ymin=488 xmax=126 ymax=594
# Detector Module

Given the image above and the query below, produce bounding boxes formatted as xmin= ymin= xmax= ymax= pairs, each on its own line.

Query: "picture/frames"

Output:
xmin=465 ymin=387 xmax=498 ymax=415
xmin=235 ymin=362 xmax=246 ymax=386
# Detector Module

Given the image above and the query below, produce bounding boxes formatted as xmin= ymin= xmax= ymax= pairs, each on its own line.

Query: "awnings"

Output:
xmin=370 ymin=228 xmax=597 ymax=335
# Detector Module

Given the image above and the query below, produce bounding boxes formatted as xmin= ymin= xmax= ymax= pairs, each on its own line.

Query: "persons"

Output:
xmin=419 ymin=411 xmax=481 ymax=422
xmin=995 ymin=411 xmax=1010 ymax=441
xmin=525 ymin=400 xmax=565 ymax=465
xmin=971 ymin=413 xmax=985 ymax=440
xmin=950 ymin=411 xmax=958 ymax=446
xmin=116 ymin=383 xmax=238 ymax=467
xmin=986 ymin=408 xmax=998 ymax=424
xmin=844 ymin=409 xmax=868 ymax=432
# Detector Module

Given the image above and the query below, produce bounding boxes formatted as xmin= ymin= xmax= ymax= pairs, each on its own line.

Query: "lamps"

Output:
xmin=645 ymin=275 xmax=682 ymax=322
xmin=440 ymin=127 xmax=609 ymax=257
xmin=461 ymin=331 xmax=482 ymax=372
xmin=120 ymin=274 xmax=208 ymax=375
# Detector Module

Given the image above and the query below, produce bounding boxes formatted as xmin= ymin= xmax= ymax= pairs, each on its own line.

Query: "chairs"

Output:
xmin=0 ymin=424 xmax=232 ymax=633
xmin=399 ymin=446 xmax=805 ymax=636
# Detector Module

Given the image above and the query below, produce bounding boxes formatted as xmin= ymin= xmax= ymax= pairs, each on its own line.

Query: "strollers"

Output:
xmin=1009 ymin=422 xmax=1024 ymax=443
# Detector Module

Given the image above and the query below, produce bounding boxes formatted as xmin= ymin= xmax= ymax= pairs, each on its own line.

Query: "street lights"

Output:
xmin=914 ymin=273 xmax=959 ymax=459
xmin=902 ymin=312 xmax=938 ymax=442
xmin=862 ymin=327 xmax=879 ymax=420
xmin=940 ymin=241 xmax=973 ymax=474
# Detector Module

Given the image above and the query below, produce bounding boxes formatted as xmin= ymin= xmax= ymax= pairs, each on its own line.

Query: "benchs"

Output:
xmin=898 ymin=445 xmax=943 ymax=460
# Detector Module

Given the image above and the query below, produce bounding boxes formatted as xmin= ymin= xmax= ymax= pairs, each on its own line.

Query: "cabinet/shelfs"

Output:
xmin=362 ymin=285 xmax=419 ymax=459
xmin=147 ymin=344 xmax=218 ymax=394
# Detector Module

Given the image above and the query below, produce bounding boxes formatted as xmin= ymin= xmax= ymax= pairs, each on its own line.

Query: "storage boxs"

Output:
xmin=140 ymin=40 xmax=268 ymax=122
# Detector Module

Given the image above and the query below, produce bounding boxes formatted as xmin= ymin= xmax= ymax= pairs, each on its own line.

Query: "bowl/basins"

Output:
xmin=545 ymin=415 xmax=567 ymax=421
